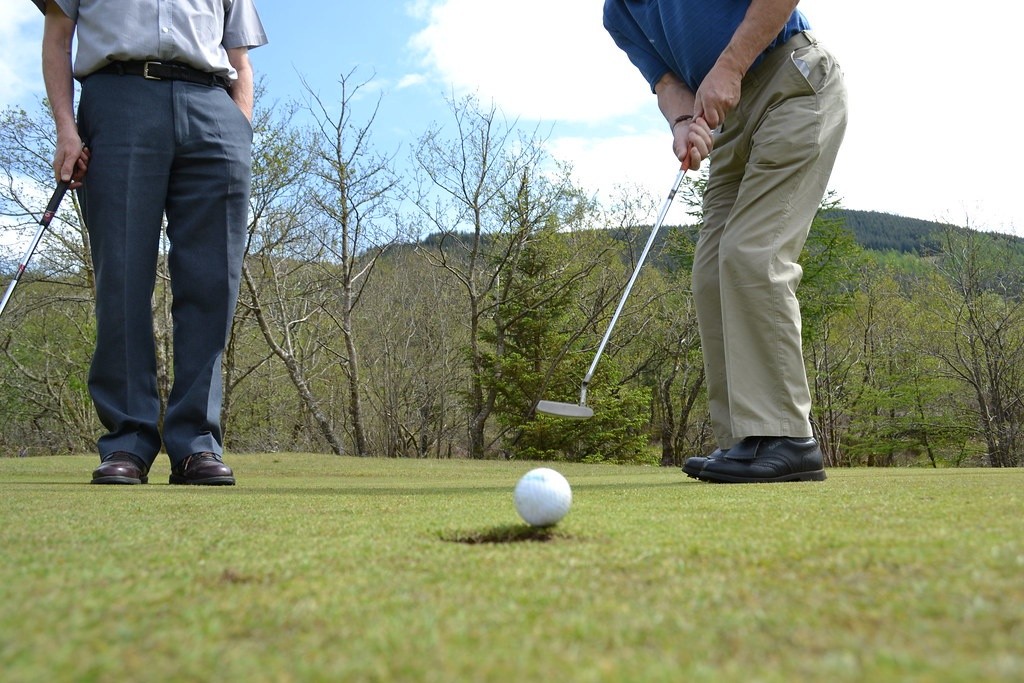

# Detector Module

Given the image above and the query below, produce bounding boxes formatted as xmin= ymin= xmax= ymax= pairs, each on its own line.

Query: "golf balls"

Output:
xmin=514 ymin=467 xmax=572 ymax=527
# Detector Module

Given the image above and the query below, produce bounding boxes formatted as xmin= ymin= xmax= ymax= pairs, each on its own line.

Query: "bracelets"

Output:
xmin=672 ymin=115 xmax=694 ymax=133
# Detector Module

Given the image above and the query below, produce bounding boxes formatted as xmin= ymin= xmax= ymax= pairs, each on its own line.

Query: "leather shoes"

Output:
xmin=168 ymin=453 xmax=236 ymax=486
xmin=698 ymin=436 xmax=827 ymax=485
xmin=91 ymin=451 xmax=148 ymax=484
xmin=681 ymin=448 xmax=729 ymax=476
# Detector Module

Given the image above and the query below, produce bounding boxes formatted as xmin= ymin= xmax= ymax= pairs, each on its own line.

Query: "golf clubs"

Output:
xmin=537 ymin=112 xmax=708 ymax=421
xmin=0 ymin=144 xmax=93 ymax=314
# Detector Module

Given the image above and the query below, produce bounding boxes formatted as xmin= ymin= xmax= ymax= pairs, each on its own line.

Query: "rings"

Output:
xmin=710 ymin=129 xmax=715 ymax=135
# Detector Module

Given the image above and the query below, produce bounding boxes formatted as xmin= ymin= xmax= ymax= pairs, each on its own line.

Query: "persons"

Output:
xmin=30 ymin=0 xmax=269 ymax=485
xmin=602 ymin=0 xmax=848 ymax=483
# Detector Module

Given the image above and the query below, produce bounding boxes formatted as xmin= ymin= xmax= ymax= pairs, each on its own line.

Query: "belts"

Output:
xmin=99 ymin=59 xmax=230 ymax=90
